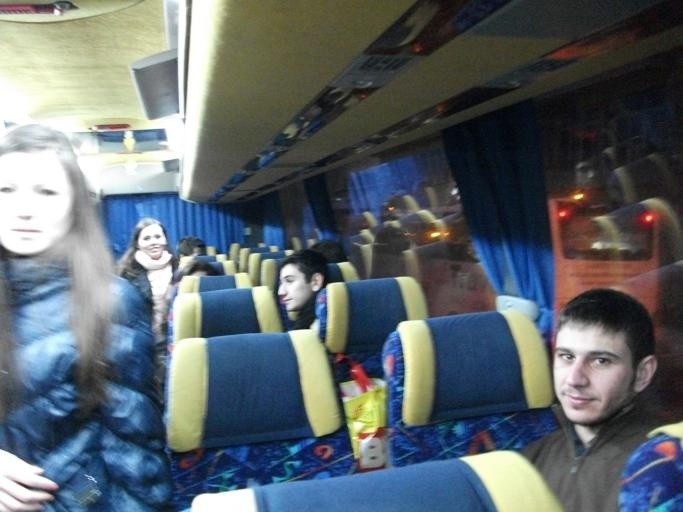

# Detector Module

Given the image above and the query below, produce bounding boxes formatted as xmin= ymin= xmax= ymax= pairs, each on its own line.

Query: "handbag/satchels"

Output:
xmin=329 ymin=354 xmax=388 ymax=472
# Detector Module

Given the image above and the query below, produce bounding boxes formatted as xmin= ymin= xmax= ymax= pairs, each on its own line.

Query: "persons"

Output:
xmin=572 ymin=150 xmax=626 ymax=220
xmin=512 ymin=287 xmax=669 ymax=511
xmin=176 ymin=236 xmax=207 ymax=258
xmin=176 ymin=260 xmax=218 ymax=277
xmin=0 ymin=123 xmax=172 ymax=510
xmin=115 ymin=216 xmax=178 ymax=417
xmin=276 ymin=248 xmax=330 ymax=330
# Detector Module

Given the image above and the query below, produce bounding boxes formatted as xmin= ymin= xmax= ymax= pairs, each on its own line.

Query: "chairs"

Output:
xmin=166 ymin=329 xmax=362 ymax=510
xmin=167 ymin=242 xmax=361 ymax=345
xmin=313 ymin=276 xmax=431 ymax=382
xmin=190 ymin=448 xmax=565 ymax=511
xmin=382 ymin=309 xmax=566 ymax=464
